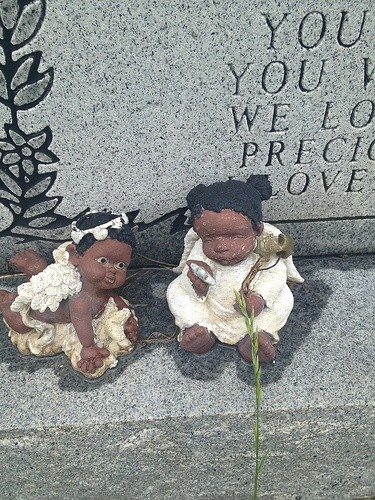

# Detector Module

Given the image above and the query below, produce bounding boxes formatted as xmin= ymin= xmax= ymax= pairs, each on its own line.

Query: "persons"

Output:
xmin=0 ymin=211 xmax=140 ymax=381
xmin=163 ymin=170 xmax=305 ymax=365
